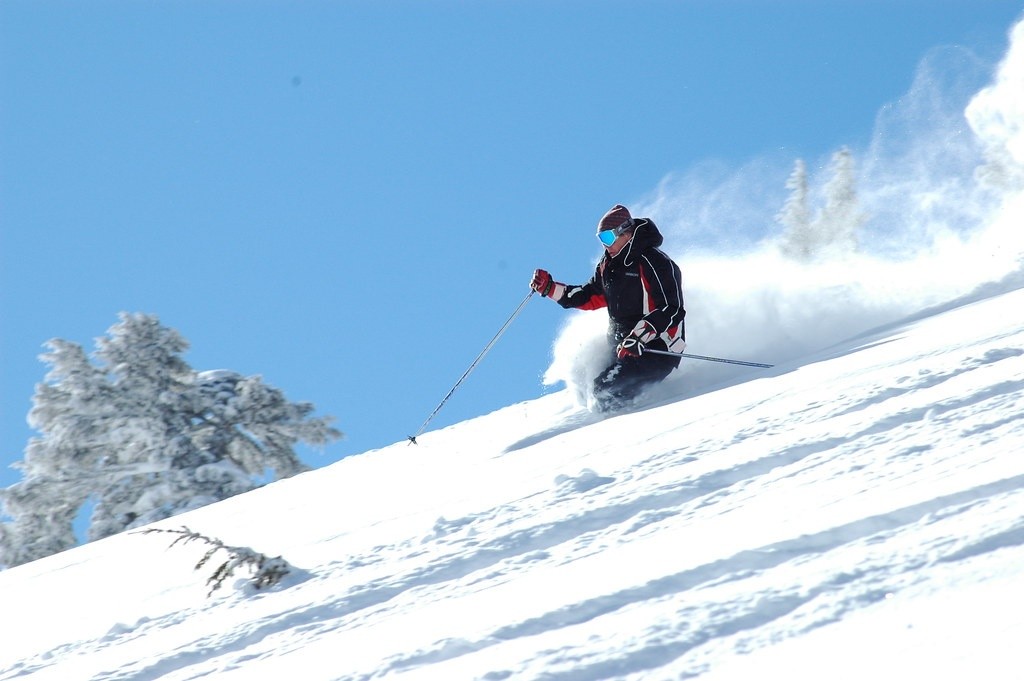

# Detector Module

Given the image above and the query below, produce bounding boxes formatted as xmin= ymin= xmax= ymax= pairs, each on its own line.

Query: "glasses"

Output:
xmin=597 ymin=218 xmax=634 ymax=247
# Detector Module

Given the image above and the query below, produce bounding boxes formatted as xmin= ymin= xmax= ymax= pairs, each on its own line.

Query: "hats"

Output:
xmin=596 ymin=205 xmax=633 ymax=234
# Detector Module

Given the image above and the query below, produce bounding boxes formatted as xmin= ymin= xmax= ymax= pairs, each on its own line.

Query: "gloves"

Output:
xmin=529 ymin=268 xmax=566 ymax=302
xmin=616 ymin=319 xmax=657 ymax=359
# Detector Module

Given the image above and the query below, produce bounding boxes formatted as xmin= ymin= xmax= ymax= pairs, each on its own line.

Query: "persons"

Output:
xmin=529 ymin=204 xmax=686 ymax=412
